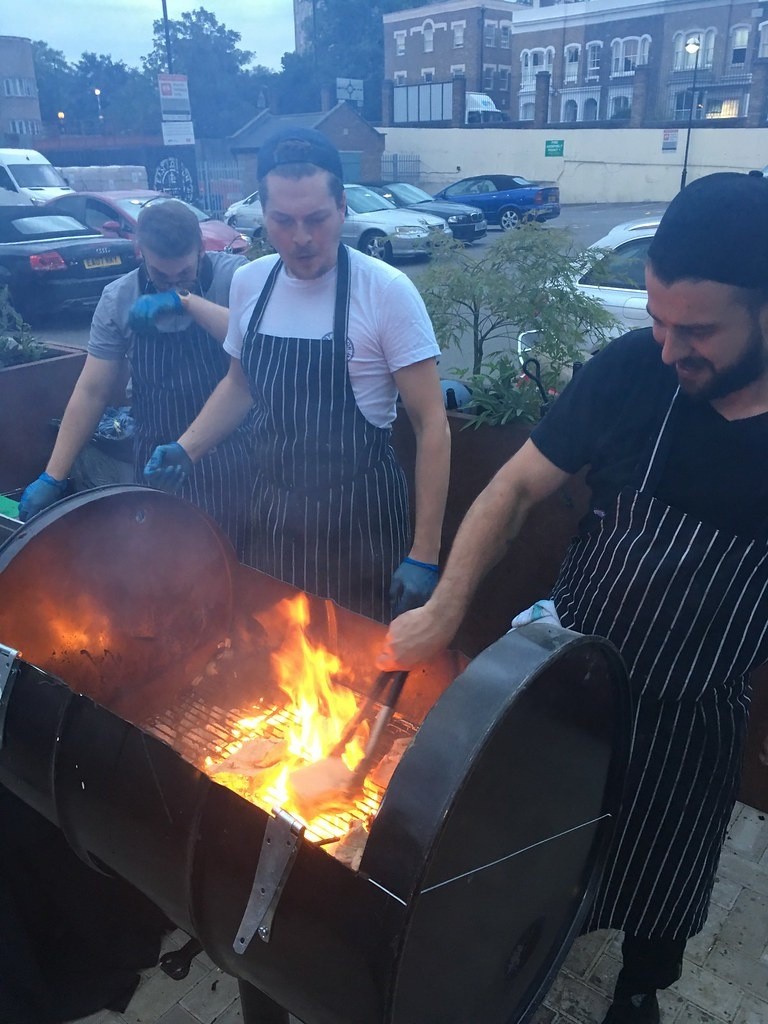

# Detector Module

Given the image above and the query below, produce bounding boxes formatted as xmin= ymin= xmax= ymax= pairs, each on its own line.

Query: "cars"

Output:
xmin=518 ymin=216 xmax=667 ymax=357
xmin=225 ymin=184 xmax=453 ymax=264
xmin=431 ymin=174 xmax=561 ymax=231
xmin=0 ymin=205 xmax=146 ymax=331
xmin=45 ymin=190 xmax=251 ymax=264
xmin=381 ymin=183 xmax=487 ymax=240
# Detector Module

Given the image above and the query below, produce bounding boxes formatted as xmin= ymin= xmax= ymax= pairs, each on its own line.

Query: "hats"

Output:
xmin=257 ymin=125 xmax=349 ymax=218
xmin=648 ymin=170 xmax=768 ymax=290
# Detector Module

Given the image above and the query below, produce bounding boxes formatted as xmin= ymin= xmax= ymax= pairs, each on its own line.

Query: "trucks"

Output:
xmin=465 ymin=92 xmax=503 ymax=124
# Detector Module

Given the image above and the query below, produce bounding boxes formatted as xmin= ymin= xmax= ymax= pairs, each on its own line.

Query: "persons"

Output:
xmin=145 ymin=127 xmax=451 ymax=625
xmin=378 ymin=170 xmax=768 ymax=1024
xmin=18 ymin=200 xmax=250 ymax=565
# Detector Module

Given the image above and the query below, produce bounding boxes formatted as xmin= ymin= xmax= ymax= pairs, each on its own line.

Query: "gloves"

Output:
xmin=143 ymin=442 xmax=195 ymax=491
xmin=389 ymin=556 xmax=439 ymax=617
xmin=129 ymin=291 xmax=183 ymax=335
xmin=18 ymin=472 xmax=67 ymax=522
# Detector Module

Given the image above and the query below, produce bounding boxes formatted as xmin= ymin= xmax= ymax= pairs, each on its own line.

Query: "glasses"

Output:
xmin=139 ymin=253 xmax=202 ymax=293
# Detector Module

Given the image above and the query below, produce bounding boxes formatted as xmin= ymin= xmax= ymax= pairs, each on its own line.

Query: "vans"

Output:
xmin=1 ymin=148 xmax=75 ymax=207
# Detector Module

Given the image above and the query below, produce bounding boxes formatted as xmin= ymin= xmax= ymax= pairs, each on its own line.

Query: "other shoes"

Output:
xmin=601 ymin=968 xmax=660 ymax=1024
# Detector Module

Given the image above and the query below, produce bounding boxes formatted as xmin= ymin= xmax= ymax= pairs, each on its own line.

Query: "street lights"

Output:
xmin=680 ymin=34 xmax=700 ymax=189
